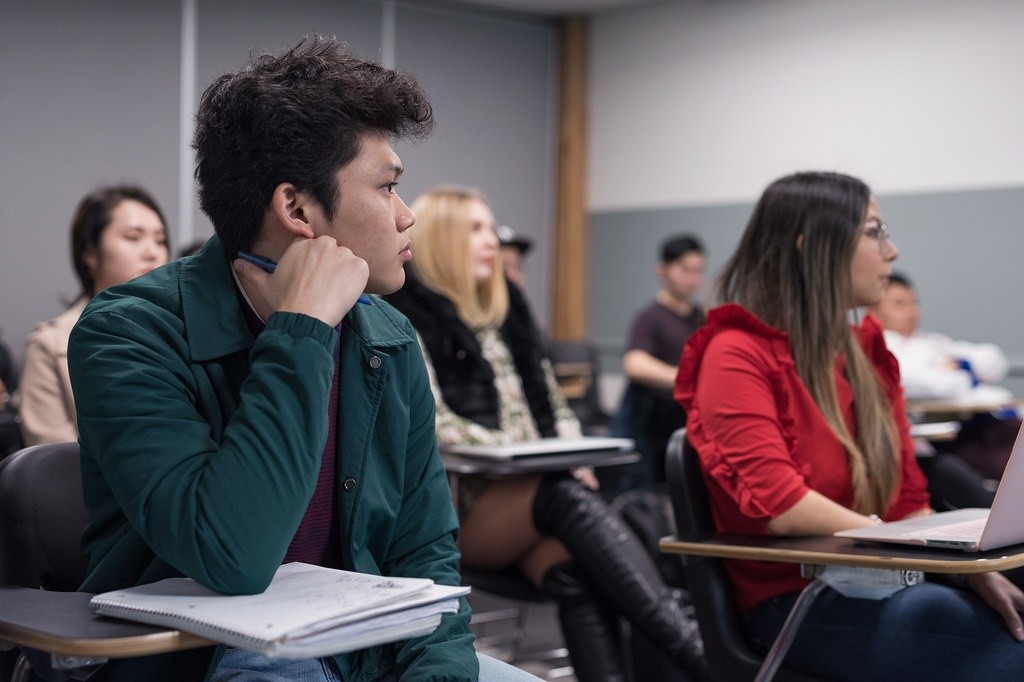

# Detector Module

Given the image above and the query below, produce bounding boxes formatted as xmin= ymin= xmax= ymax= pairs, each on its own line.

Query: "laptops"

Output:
xmin=833 ymin=420 xmax=1024 ymax=552
xmin=446 ymin=437 xmax=635 ymax=464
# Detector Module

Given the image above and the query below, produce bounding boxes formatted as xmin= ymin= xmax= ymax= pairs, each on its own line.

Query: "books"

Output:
xmin=90 ymin=560 xmax=472 ymax=659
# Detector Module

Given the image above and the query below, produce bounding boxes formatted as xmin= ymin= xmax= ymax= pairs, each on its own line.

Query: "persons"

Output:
xmin=376 ymin=187 xmax=706 ymax=682
xmin=70 ymin=31 xmax=547 ymax=682
xmin=674 ymin=171 xmax=1024 ymax=682
xmin=0 ymin=183 xmax=1019 ymax=509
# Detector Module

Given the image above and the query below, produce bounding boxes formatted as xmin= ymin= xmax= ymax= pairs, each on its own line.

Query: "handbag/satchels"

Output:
xmin=810 ymin=559 xmax=925 ymax=601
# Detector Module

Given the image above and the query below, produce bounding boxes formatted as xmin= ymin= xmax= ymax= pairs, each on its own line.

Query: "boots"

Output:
xmin=530 ymin=469 xmax=706 ymax=671
xmin=538 ymin=551 xmax=629 ymax=682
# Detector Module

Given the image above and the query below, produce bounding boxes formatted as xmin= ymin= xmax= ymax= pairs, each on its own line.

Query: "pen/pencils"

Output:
xmin=235 ymin=252 xmax=375 ymax=306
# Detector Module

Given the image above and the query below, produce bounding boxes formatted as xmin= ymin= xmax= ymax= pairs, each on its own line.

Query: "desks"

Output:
xmin=0 ymin=586 xmax=217 ymax=681
xmin=658 ymin=531 xmax=1024 ymax=682
xmin=443 ymin=448 xmax=644 ymax=511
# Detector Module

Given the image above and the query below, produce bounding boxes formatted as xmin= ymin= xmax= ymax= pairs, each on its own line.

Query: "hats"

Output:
xmin=497 ymin=226 xmax=533 ymax=255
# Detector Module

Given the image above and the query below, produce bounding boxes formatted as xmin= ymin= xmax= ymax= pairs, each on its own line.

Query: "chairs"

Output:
xmin=0 ymin=443 xmax=97 ymax=590
xmin=664 ymin=428 xmax=809 ymax=682
xmin=549 ymin=341 xmax=627 ymax=436
